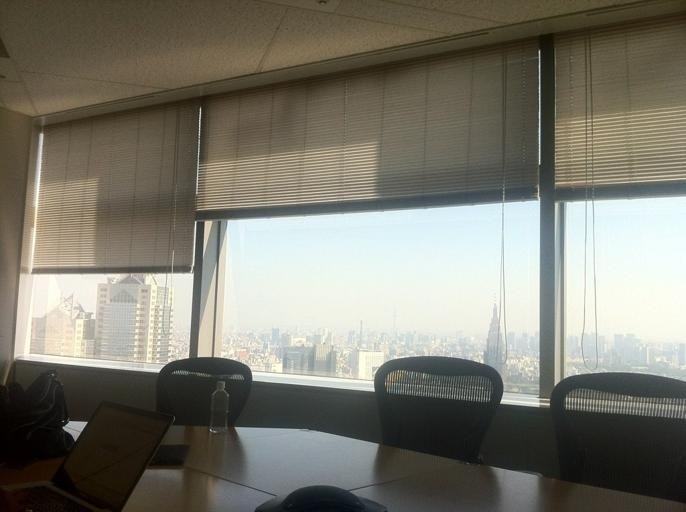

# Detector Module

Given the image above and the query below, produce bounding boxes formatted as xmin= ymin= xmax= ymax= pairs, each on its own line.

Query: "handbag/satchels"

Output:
xmin=1 ymin=369 xmax=67 ymax=431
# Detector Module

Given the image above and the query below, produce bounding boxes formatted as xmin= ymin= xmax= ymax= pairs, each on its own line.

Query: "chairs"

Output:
xmin=549 ymin=372 xmax=686 ymax=503
xmin=156 ymin=357 xmax=253 ymax=426
xmin=375 ymin=356 xmax=504 ymax=465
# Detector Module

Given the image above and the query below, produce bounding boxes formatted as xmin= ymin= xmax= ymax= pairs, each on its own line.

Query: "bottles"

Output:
xmin=207 ymin=381 xmax=229 ymax=433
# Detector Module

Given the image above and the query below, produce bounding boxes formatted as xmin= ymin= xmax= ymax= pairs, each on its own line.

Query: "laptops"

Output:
xmin=0 ymin=401 xmax=176 ymax=512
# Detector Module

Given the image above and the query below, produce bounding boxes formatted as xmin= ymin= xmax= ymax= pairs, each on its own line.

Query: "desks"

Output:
xmin=1 ymin=421 xmax=685 ymax=512
